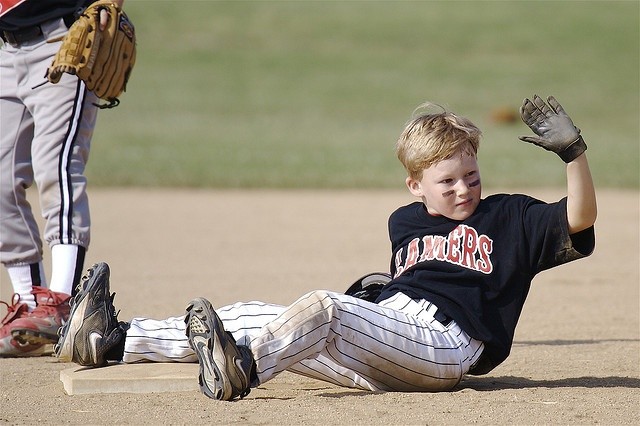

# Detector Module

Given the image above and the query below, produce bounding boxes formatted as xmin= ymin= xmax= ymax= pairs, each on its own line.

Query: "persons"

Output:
xmin=1 ymin=1 xmax=137 ymax=357
xmin=55 ymin=93 xmax=598 ymax=400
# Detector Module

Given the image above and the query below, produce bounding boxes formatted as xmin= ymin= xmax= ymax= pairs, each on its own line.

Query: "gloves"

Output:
xmin=518 ymin=94 xmax=587 ymax=163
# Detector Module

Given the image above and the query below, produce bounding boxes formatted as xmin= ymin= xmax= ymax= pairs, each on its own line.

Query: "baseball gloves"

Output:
xmin=46 ymin=1 xmax=137 ymax=111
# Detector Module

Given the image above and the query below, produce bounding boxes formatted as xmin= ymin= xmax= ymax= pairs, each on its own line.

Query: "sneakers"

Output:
xmin=184 ymin=297 xmax=252 ymax=399
xmin=11 ymin=286 xmax=72 ymax=343
xmin=1 ymin=293 xmax=46 ymax=357
xmin=55 ymin=262 xmax=125 ymax=370
